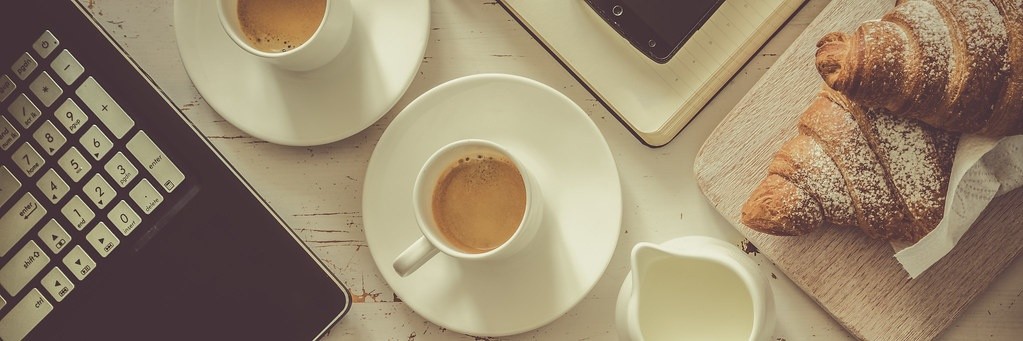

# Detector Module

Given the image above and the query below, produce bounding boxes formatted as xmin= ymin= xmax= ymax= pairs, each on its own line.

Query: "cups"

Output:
xmin=216 ymin=0 xmax=355 ymax=71
xmin=615 ymin=234 xmax=776 ymax=341
xmin=392 ymin=139 xmax=544 ymax=277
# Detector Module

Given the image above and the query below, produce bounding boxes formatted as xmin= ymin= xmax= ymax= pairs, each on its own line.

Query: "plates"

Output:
xmin=362 ymin=74 xmax=622 ymax=337
xmin=172 ymin=0 xmax=431 ymax=146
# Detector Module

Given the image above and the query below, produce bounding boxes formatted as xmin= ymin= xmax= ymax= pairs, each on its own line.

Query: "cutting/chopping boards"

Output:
xmin=694 ymin=0 xmax=1023 ymax=341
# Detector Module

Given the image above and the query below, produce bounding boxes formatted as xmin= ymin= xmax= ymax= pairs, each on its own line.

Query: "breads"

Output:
xmin=739 ymin=84 xmax=956 ymax=243
xmin=813 ymin=0 xmax=1023 ymax=142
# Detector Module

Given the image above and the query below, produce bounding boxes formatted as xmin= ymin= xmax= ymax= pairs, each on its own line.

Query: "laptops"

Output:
xmin=0 ymin=0 xmax=351 ymax=341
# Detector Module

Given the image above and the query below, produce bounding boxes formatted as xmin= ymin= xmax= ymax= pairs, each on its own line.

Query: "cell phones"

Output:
xmin=584 ymin=0 xmax=726 ymax=65
